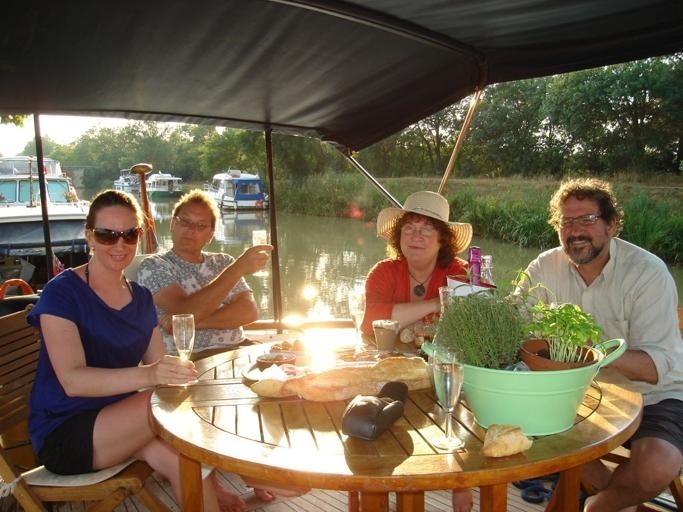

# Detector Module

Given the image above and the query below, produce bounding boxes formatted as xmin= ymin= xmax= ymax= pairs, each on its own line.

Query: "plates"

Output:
xmin=255 ymin=353 xmax=295 ymax=371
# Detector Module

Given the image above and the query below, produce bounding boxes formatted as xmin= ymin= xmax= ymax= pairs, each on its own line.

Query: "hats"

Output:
xmin=376 ymin=189 xmax=474 ymax=254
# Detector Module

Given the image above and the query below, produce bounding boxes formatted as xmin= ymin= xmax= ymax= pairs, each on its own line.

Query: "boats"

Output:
xmin=148 ymin=198 xmax=178 ymax=225
xmin=202 ymin=166 xmax=268 ymax=211
xmin=146 ymin=170 xmax=185 ymax=198
xmin=0 ymin=156 xmax=98 ymax=270
xmin=111 ymin=167 xmax=139 ymax=194
xmin=212 ymin=209 xmax=268 ymax=250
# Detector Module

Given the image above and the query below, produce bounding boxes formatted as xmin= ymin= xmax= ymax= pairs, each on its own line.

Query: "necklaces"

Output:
xmin=408 ymin=271 xmax=433 ymax=296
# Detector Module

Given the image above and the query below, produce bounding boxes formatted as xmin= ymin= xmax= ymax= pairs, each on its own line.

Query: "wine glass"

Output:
xmin=252 ymin=231 xmax=272 ymax=279
xmin=432 ymin=348 xmax=465 ymax=450
xmin=170 ymin=315 xmax=197 ymax=389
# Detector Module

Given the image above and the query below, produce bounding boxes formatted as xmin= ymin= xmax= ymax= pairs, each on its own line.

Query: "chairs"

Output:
xmin=0 ymin=304 xmax=169 ymax=511
xmin=124 ymin=254 xmax=304 ymax=365
xmin=546 ymin=308 xmax=683 ymax=512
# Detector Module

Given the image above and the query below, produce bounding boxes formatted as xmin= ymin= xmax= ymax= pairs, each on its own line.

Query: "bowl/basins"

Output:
xmin=424 ymin=335 xmax=632 ymax=437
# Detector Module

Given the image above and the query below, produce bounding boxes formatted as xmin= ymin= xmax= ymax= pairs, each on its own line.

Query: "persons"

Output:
xmin=510 ymin=180 xmax=683 ymax=512
xmin=360 ymin=191 xmax=474 ymax=512
xmin=137 ymin=189 xmax=278 ymax=512
xmin=25 ymin=191 xmax=312 ymax=512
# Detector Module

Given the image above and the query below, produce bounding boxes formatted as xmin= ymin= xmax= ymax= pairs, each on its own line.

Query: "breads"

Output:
xmin=282 ymin=356 xmax=431 ymax=402
xmin=478 ymin=423 xmax=534 ymax=459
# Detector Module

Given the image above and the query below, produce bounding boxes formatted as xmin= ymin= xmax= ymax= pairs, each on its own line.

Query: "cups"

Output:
xmin=414 ymin=320 xmax=432 ymax=349
xmin=372 ymin=318 xmax=399 ymax=359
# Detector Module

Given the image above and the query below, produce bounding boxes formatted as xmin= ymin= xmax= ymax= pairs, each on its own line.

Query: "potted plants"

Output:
xmin=518 ymin=300 xmax=608 ymax=370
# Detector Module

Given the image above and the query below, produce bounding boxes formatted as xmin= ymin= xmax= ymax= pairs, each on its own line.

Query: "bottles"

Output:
xmin=433 ymin=286 xmax=451 ymax=347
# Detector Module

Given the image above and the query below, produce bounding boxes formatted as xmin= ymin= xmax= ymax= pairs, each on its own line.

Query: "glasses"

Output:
xmin=556 ymin=214 xmax=600 ymax=229
xmin=90 ymin=227 xmax=140 ymax=245
